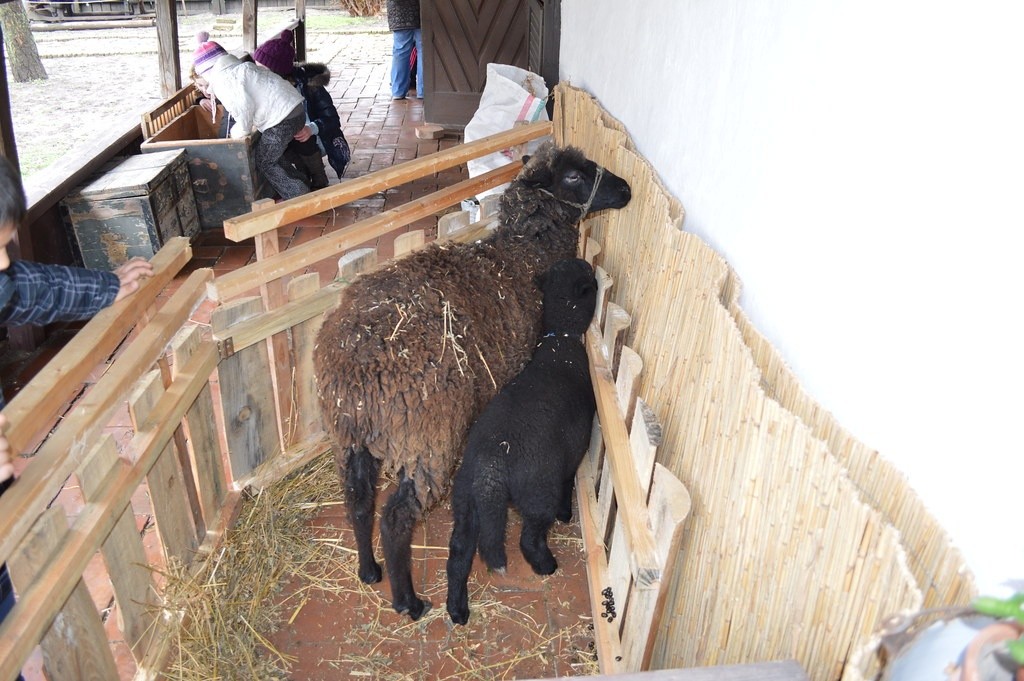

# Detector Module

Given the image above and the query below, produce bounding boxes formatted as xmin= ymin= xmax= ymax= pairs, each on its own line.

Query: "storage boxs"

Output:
xmin=59 ymin=148 xmax=203 ymax=269
xmin=139 ymin=105 xmax=270 ymax=228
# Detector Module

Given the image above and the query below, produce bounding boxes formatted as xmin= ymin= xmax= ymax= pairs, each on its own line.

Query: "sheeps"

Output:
xmin=308 ymin=139 xmax=632 ymax=620
xmin=443 ymin=257 xmax=600 ymax=625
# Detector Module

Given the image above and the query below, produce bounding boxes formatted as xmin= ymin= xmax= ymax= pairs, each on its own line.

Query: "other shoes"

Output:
xmin=393 ymin=96 xmax=404 ymax=100
xmin=276 ymin=198 xmax=283 ymax=204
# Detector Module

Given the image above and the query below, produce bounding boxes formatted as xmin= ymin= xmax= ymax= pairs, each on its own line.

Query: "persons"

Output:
xmin=387 ymin=0 xmax=424 ymax=100
xmin=0 ymin=161 xmax=155 ymax=680
xmin=190 ymin=30 xmax=352 ymax=201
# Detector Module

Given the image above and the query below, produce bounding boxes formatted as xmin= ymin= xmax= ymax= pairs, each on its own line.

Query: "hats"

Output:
xmin=193 ymin=32 xmax=227 ymax=75
xmin=254 ymin=29 xmax=295 ymax=78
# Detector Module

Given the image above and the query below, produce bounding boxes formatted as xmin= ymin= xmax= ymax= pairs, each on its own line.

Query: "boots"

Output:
xmin=284 ymin=150 xmax=311 ymax=180
xmin=299 ymin=149 xmax=328 ymax=189
